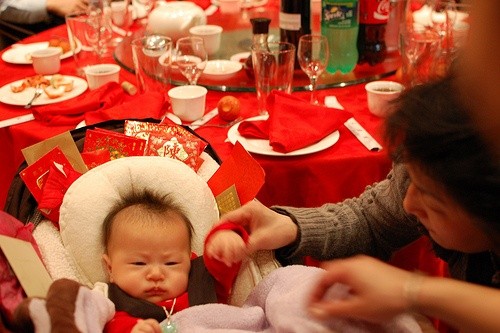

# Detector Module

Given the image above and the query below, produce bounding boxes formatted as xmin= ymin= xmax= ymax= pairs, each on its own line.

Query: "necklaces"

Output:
xmin=162 ymin=298 xmax=176 ymax=333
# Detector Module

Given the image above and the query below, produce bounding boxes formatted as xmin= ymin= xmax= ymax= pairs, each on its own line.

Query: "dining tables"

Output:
xmin=0 ymin=0 xmax=451 ymax=333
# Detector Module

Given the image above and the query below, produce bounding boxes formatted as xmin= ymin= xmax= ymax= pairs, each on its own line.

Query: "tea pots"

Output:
xmin=147 ymin=0 xmax=208 ymax=43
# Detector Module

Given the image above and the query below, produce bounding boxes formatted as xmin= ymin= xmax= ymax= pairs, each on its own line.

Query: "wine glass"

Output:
xmin=174 ymin=36 xmax=207 ymax=84
xmin=297 ymin=34 xmax=330 ymax=107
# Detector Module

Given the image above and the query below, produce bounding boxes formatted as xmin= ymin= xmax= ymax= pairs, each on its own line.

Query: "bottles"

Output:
xmin=357 ymin=0 xmax=390 ymax=66
xmin=279 ymin=0 xmax=313 ymax=69
xmin=243 ymin=17 xmax=277 ymax=82
xmin=320 ymin=0 xmax=358 ymax=75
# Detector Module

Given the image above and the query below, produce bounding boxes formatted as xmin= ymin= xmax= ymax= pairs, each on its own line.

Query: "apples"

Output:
xmin=218 ymin=96 xmax=240 ymax=121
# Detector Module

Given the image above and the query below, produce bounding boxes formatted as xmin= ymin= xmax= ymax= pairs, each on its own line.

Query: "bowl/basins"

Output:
xmin=197 ymin=60 xmax=243 ymax=80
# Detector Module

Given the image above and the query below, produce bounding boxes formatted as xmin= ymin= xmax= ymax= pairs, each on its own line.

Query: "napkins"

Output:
xmin=34 ymin=79 xmax=165 ymax=129
xmin=238 ymin=89 xmax=353 ymax=154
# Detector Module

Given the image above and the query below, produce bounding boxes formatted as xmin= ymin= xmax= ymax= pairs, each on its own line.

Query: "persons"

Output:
xmin=0 ymin=0 xmax=90 ymax=52
xmin=102 ymin=188 xmax=250 ymax=333
xmin=212 ymin=0 xmax=500 ymax=333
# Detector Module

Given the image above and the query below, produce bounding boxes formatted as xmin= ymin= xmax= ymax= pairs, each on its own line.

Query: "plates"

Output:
xmin=227 ymin=115 xmax=339 ymax=156
xmin=1 ymin=41 xmax=81 ymax=64
xmin=0 ymin=74 xmax=88 ymax=106
xmin=230 ymin=52 xmax=267 ymax=66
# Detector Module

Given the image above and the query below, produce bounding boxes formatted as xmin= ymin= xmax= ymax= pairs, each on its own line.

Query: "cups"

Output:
xmin=365 ymin=80 xmax=405 ymax=117
xmin=190 ymin=25 xmax=223 ymax=56
xmin=29 ymin=46 xmax=62 ymax=75
xmin=445 ymin=4 xmax=470 ymax=65
xmin=385 ymin=2 xmax=399 ymax=51
xmin=250 ymin=42 xmax=296 ymax=100
xmin=400 ymin=0 xmax=455 ymax=88
xmin=167 ymin=85 xmax=208 ymax=121
xmin=64 ymin=0 xmax=172 ymax=107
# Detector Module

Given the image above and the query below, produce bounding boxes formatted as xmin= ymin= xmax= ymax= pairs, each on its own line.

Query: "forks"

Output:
xmin=26 ymin=84 xmax=43 ymax=107
